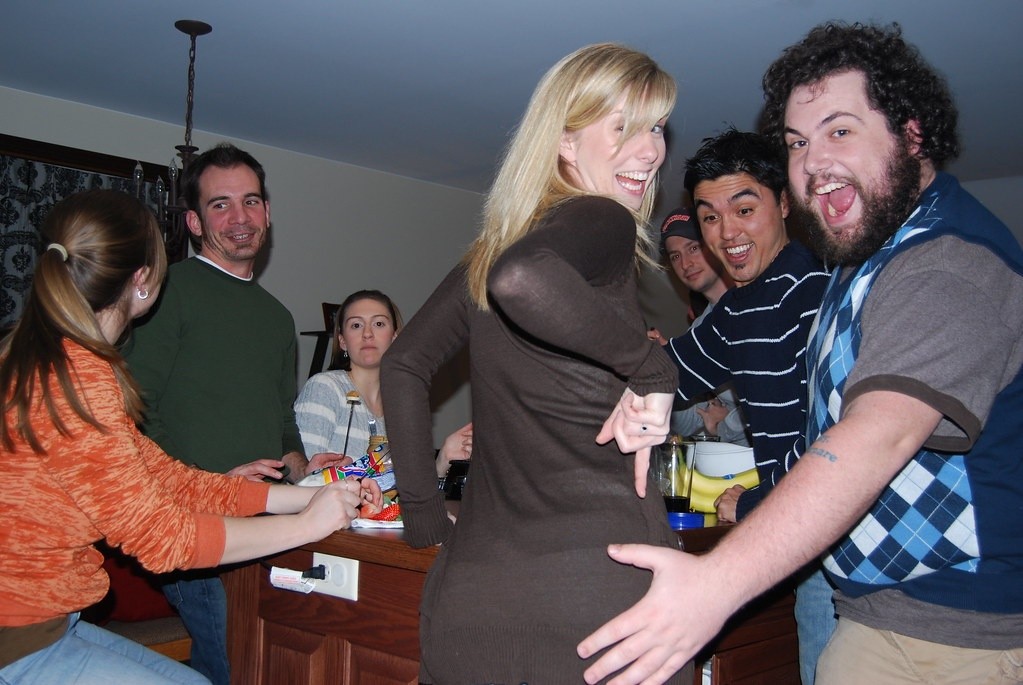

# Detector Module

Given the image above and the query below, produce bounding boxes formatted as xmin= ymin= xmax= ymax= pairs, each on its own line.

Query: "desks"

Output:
xmin=82 ymin=475 xmax=805 ymax=685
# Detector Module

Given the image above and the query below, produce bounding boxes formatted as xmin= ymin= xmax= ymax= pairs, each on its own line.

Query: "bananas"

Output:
xmin=671 ymin=436 xmax=760 ymax=514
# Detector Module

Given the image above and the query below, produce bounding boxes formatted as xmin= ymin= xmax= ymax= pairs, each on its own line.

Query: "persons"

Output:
xmin=666 ymin=210 xmax=750 ymax=443
xmin=374 ymin=42 xmax=699 ymax=683
xmin=292 ymin=291 xmax=472 ymax=492
xmin=582 ymin=17 xmax=1023 ymax=685
xmin=0 ymin=191 xmax=394 ymax=685
xmin=642 ymin=130 xmax=846 ymax=685
xmin=107 ymin=139 xmax=358 ymax=685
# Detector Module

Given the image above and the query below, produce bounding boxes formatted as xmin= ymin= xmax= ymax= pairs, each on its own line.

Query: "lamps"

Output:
xmin=126 ymin=19 xmax=213 ymax=262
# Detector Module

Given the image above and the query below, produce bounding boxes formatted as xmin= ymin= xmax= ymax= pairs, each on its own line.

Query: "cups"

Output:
xmin=648 ymin=441 xmax=697 ymax=513
xmin=690 ymin=435 xmax=720 ymax=442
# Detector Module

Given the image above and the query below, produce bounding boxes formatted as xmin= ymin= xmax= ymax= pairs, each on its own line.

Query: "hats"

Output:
xmin=660 ymin=208 xmax=704 ymax=245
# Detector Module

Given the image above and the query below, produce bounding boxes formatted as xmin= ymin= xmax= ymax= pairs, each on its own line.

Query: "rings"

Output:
xmin=342 ymin=480 xmax=350 ymax=490
